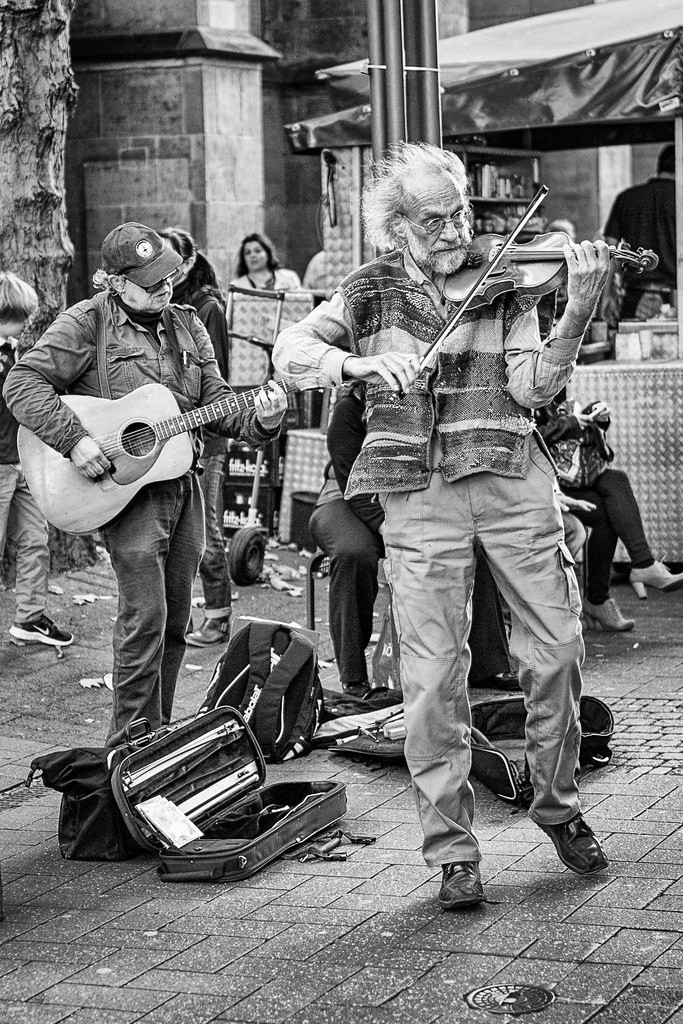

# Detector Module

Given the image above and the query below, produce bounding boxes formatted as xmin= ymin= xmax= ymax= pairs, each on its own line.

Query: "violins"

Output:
xmin=442 ymin=231 xmax=658 ymax=309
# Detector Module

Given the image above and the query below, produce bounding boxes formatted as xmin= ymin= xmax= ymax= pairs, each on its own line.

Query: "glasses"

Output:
xmin=401 ymin=203 xmax=474 ymax=236
xmin=123 ymin=268 xmax=179 ymax=294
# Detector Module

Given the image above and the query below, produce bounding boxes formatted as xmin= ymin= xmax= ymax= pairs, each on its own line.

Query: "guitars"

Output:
xmin=16 ymin=372 xmax=343 ymax=534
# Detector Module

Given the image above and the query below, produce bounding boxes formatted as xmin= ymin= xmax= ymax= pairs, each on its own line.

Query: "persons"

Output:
xmin=272 ymin=142 xmax=618 ymax=907
xmin=0 ymin=144 xmax=683 ymax=748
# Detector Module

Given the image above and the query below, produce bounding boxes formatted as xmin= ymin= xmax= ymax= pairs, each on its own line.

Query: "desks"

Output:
xmin=566 ymin=360 xmax=683 ymax=563
xmin=277 ymin=429 xmax=332 ymax=543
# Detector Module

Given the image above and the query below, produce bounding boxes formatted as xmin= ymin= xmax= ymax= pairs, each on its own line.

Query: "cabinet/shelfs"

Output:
xmin=443 ymin=145 xmax=541 ymax=241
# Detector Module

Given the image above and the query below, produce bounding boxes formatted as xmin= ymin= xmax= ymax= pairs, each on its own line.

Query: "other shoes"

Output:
xmin=470 ymin=672 xmax=523 ymax=691
xmin=342 ymin=680 xmax=371 ymax=695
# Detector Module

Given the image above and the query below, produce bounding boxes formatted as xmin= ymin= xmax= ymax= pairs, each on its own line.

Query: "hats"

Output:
xmin=100 ymin=221 xmax=184 ymax=287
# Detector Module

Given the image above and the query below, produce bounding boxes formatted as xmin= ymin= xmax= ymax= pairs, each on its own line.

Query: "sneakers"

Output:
xmin=8 ymin=614 xmax=74 ymax=646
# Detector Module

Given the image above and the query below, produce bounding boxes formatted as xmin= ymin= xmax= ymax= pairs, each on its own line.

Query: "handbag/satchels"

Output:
xmin=26 ymin=747 xmax=145 ymax=862
xmin=549 ymin=397 xmax=608 ymax=489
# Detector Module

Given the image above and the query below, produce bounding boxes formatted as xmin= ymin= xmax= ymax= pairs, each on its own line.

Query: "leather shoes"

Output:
xmin=185 ymin=615 xmax=231 ymax=647
xmin=438 ymin=861 xmax=483 ymax=910
xmin=531 ymin=811 xmax=609 ymax=875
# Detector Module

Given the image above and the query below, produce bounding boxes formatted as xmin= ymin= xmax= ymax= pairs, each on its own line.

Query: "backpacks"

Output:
xmin=197 ymin=621 xmax=325 ymax=763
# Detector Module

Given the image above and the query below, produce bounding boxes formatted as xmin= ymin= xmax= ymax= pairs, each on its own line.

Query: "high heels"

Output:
xmin=582 ymin=597 xmax=635 ymax=631
xmin=630 ymin=555 xmax=683 ymax=600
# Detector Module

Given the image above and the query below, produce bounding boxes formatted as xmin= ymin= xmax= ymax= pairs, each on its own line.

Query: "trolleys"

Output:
xmin=223 ymin=282 xmax=287 ymax=587
